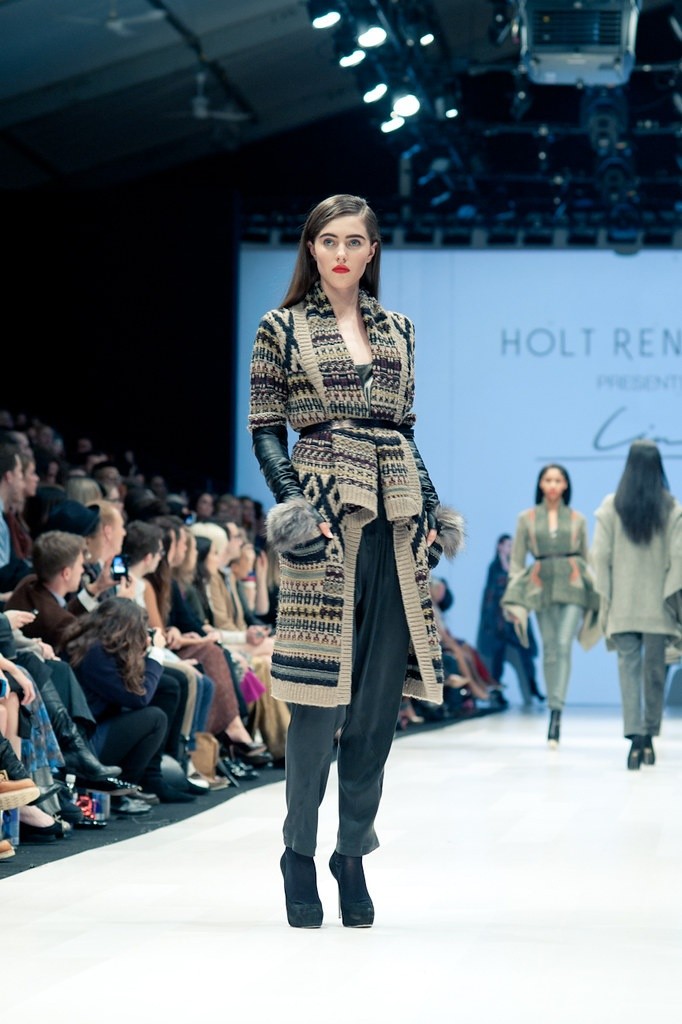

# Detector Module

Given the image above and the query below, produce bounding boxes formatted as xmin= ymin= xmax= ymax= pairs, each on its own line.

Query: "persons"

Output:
xmin=0 ymin=406 xmax=496 ymax=859
xmin=499 ymin=463 xmax=598 ymax=748
xmin=591 ymin=439 xmax=682 ymax=770
xmin=245 ymin=194 xmax=470 ymax=928
xmin=476 ymin=536 xmax=546 ymax=703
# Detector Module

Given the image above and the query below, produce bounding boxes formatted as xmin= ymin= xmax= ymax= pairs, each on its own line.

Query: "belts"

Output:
xmin=299 ymin=417 xmax=399 ymax=438
xmin=534 ymin=552 xmax=581 ymax=560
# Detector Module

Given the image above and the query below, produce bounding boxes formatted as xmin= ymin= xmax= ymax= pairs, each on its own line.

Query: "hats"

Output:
xmin=44 ymin=501 xmax=99 ymax=537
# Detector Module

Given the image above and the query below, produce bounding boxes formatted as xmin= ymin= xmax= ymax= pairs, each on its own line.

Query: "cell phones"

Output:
xmin=113 ymin=555 xmax=129 ymax=583
xmin=31 ymin=610 xmax=39 ymax=615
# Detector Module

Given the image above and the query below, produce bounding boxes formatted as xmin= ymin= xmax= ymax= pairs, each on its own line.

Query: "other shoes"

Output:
xmin=443 ymin=672 xmax=470 ymax=689
xmin=0 ymin=679 xmax=272 ymax=859
xmin=546 ymin=708 xmax=560 ymax=743
xmin=401 ymin=696 xmax=426 ymax=722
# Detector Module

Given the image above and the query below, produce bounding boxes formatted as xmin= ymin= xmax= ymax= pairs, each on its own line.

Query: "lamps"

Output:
xmin=304 ymin=0 xmax=420 ymax=136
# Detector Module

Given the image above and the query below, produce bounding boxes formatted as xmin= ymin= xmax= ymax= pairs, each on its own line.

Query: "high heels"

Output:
xmin=628 ymin=736 xmax=645 ymax=771
xmin=642 ymin=738 xmax=655 ymax=766
xmin=329 ymin=850 xmax=374 ymax=929
xmin=280 ymin=848 xmax=324 ymax=927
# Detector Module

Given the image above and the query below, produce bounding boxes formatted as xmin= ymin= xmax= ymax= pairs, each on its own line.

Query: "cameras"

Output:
xmin=143 ymin=629 xmax=155 ymax=650
